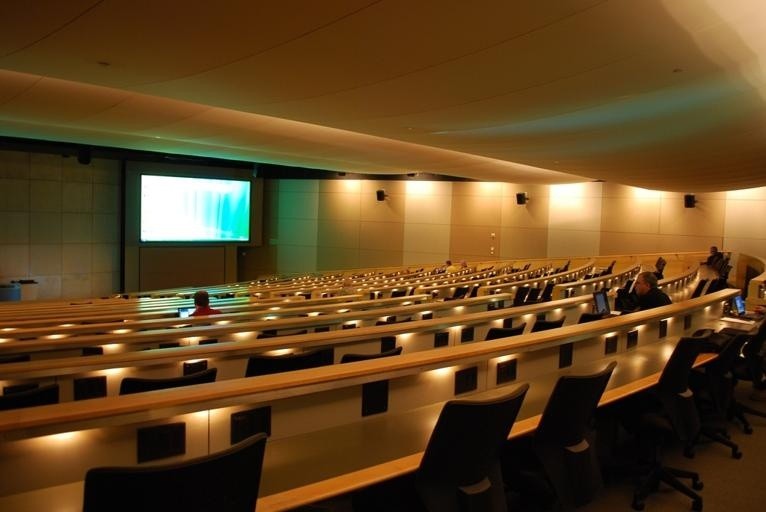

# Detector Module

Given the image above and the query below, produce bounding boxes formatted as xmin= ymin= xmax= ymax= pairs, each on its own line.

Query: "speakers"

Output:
xmin=684 ymin=195 xmax=694 ymax=208
xmin=377 ymin=191 xmax=384 ymax=201
xmin=517 ymin=193 xmax=525 ymax=205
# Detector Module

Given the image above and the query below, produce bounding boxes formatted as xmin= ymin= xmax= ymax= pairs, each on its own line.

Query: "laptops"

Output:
xmin=593 ymin=291 xmax=617 ymax=318
xmin=178 ymin=307 xmax=196 ymax=318
xmin=735 ymin=295 xmax=764 ymax=320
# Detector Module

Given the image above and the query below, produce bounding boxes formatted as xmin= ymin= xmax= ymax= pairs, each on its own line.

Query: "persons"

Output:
xmin=700 ymin=245 xmax=723 ymax=265
xmin=188 ymin=290 xmax=223 ymax=318
xmin=460 ymin=260 xmax=468 ymax=269
xmin=445 ymin=260 xmax=458 ymax=273
xmin=752 ymin=304 xmax=766 ymax=316
xmin=634 ymin=271 xmax=672 ymax=312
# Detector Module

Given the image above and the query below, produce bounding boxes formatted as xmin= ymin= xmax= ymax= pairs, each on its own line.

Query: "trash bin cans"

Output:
xmin=1 ymin=285 xmax=22 ymax=300
